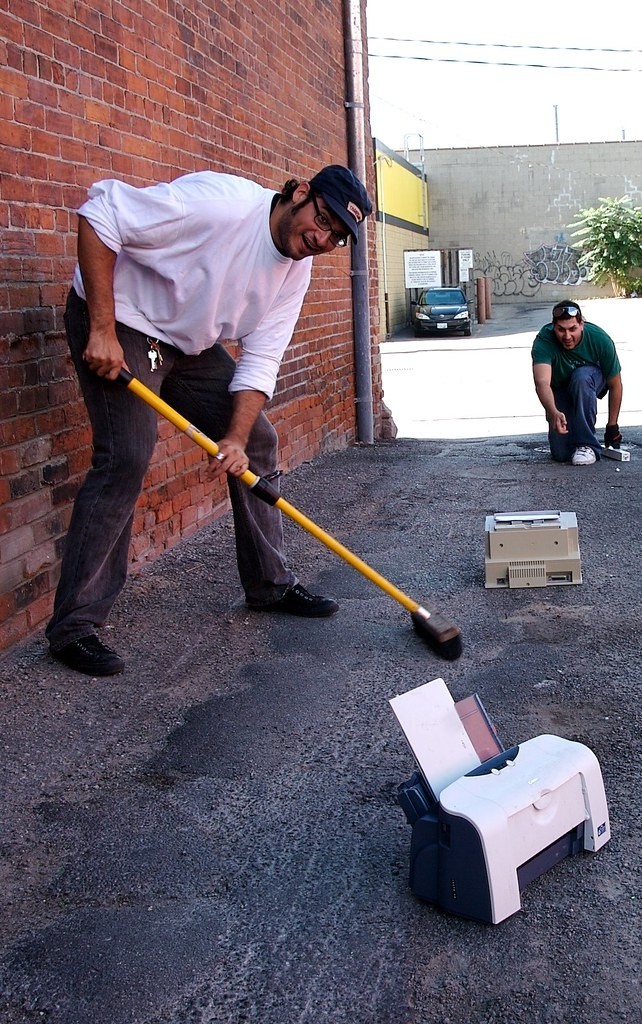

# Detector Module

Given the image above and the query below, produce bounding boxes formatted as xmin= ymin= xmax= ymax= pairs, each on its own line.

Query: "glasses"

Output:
xmin=552 ymin=306 xmax=580 ymax=317
xmin=309 ymin=190 xmax=348 ymax=248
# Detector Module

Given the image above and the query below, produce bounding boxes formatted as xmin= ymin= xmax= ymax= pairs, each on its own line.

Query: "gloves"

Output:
xmin=604 ymin=424 xmax=622 ymax=449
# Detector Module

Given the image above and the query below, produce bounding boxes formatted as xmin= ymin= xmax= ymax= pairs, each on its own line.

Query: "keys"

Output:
xmin=145 ymin=336 xmax=163 ymax=372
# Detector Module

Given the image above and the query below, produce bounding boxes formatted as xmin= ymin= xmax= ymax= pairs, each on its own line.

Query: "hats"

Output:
xmin=311 ymin=165 xmax=373 ymax=246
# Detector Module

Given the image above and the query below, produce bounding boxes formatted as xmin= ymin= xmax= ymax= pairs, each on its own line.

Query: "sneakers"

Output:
xmin=572 ymin=446 xmax=596 ymax=465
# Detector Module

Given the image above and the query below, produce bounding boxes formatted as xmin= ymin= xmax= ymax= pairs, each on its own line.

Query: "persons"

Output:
xmin=530 ymin=299 xmax=624 ymax=466
xmin=42 ymin=162 xmax=374 ymax=679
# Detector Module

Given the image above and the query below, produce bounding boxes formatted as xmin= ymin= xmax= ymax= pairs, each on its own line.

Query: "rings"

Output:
xmin=215 ymin=452 xmax=225 ymax=461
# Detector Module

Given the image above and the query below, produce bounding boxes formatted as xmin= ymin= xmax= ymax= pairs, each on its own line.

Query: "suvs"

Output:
xmin=410 ymin=286 xmax=474 ymax=337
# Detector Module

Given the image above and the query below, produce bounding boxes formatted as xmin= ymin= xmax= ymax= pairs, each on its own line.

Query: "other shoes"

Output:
xmin=250 ymin=583 xmax=339 ymax=615
xmin=49 ymin=635 xmax=126 ymax=677
